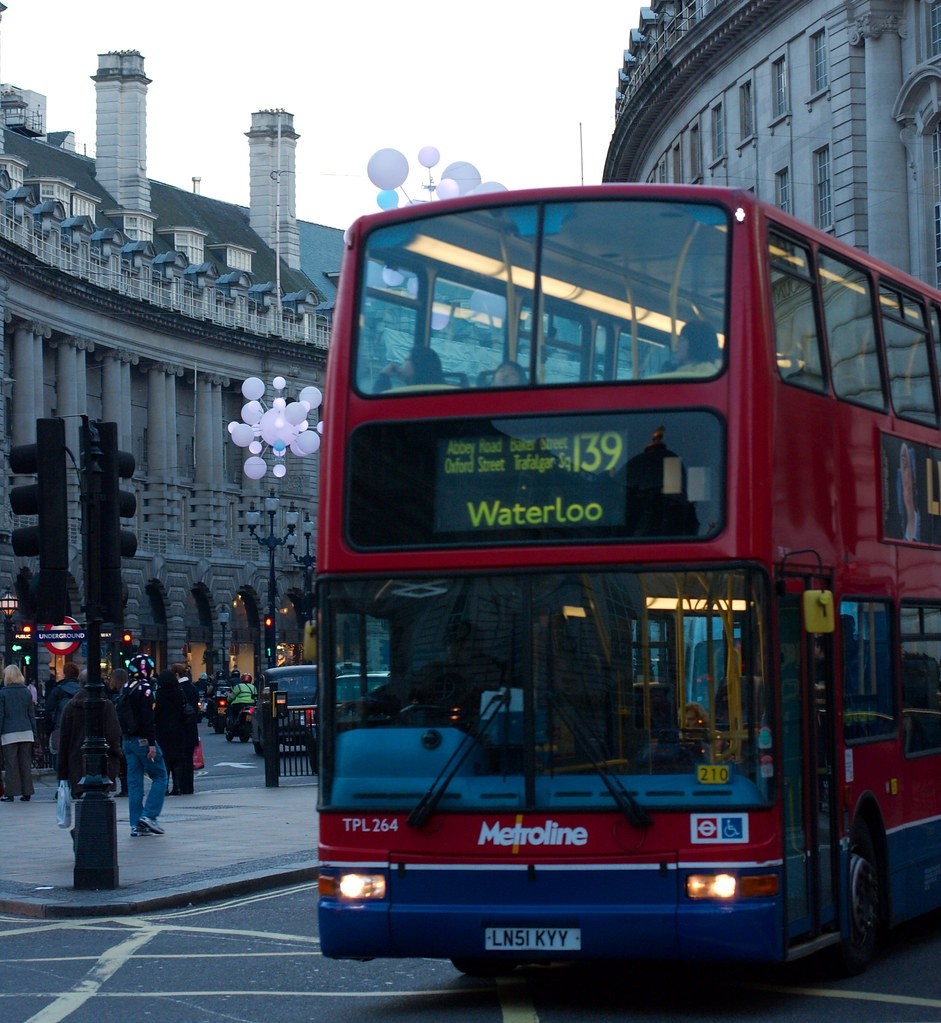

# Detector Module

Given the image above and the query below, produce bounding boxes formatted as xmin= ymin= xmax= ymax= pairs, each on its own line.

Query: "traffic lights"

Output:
xmin=80 ymin=423 xmax=138 ymax=623
xmin=11 ymin=418 xmax=68 ymax=625
xmin=123 ymin=631 xmax=133 ymax=647
xmin=11 ymin=626 xmax=34 ymax=667
xmin=263 ymin=614 xmax=273 ymax=658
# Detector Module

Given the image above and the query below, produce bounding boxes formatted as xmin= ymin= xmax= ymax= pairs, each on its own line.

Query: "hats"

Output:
xmin=200 ymin=673 xmax=207 ymax=679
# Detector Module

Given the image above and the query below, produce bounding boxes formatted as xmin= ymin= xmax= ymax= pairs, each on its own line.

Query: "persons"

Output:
xmin=190 ymin=670 xmax=260 ymax=731
xmin=56 ymin=669 xmax=123 ymax=853
xmin=0 ymin=662 xmax=199 ymax=802
xmin=491 ymin=359 xmax=525 ymax=387
xmin=678 ymin=614 xmax=863 ymax=753
xmin=371 ymin=346 xmax=444 ymax=393
xmin=660 ymin=320 xmax=725 ymax=375
xmin=355 ymin=609 xmax=612 ymax=768
xmin=119 ymin=655 xmax=169 ymax=837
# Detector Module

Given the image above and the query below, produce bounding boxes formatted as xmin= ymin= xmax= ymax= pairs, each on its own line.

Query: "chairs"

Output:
xmin=660 ymin=320 xmax=723 ymax=374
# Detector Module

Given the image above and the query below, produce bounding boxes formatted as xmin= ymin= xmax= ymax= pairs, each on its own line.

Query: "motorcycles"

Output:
xmin=197 ymin=688 xmax=254 ymax=743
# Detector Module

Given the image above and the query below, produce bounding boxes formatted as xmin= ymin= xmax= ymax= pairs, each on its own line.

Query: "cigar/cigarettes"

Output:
xmin=151 ymin=757 xmax=154 ymax=762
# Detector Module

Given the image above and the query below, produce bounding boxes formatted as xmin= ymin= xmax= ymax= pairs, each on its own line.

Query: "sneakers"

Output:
xmin=130 ymin=816 xmax=164 ymax=836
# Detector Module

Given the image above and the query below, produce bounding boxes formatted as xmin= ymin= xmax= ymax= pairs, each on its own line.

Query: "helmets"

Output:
xmin=216 ymin=671 xmax=227 ymax=679
xmin=240 ymin=673 xmax=252 ymax=682
xmin=231 ymin=669 xmax=240 ymax=678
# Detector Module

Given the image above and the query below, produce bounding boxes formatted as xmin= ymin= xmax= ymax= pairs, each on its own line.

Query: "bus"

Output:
xmin=311 ymin=183 xmax=940 ymax=985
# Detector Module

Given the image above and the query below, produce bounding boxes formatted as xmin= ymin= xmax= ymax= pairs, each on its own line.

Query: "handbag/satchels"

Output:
xmin=56 ymin=780 xmax=72 ymax=828
xmin=193 ymin=737 xmax=204 ymax=770
xmin=179 ymin=685 xmax=194 ymax=725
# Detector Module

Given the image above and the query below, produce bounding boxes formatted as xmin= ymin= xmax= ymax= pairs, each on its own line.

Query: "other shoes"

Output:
xmin=20 ymin=795 xmax=31 ymax=801
xmin=0 ymin=795 xmax=15 ymax=802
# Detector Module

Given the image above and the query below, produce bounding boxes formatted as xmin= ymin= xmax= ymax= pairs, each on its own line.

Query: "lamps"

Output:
xmin=383 ymin=265 xmax=507 ymax=330
xmin=368 ymin=146 xmax=510 ymax=210
xmin=227 ymin=375 xmax=324 ymax=481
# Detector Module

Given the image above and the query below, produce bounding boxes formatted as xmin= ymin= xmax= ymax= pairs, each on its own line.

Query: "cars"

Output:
xmin=251 ymin=664 xmax=320 ymax=752
xmin=307 ymin=669 xmax=392 ymax=772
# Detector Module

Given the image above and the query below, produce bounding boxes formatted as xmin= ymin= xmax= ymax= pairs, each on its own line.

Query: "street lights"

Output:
xmin=245 ymin=489 xmax=300 ymax=667
xmin=217 ymin=605 xmax=230 ymax=673
xmin=289 ymin=514 xmax=317 ymax=622
